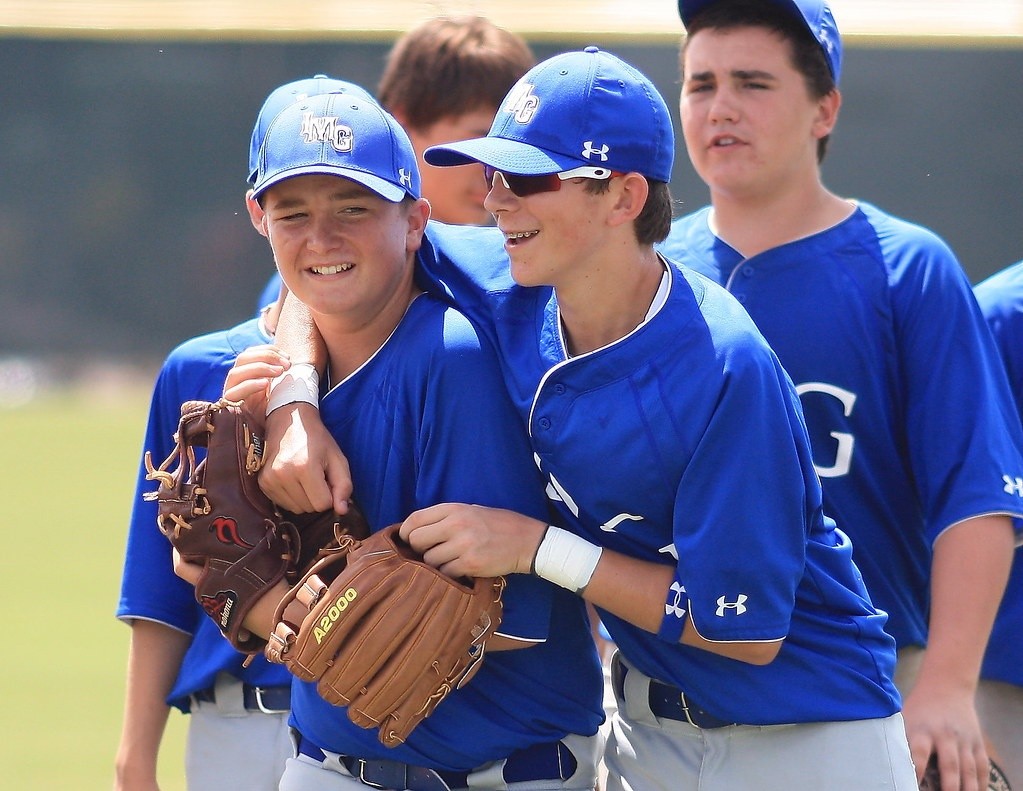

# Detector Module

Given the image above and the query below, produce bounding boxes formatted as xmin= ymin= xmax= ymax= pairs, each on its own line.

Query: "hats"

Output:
xmin=678 ymin=0 xmax=841 ymax=87
xmin=423 ymin=46 xmax=674 ymax=184
xmin=250 ymin=92 xmax=423 ymax=204
xmin=246 ymin=72 xmax=382 ymax=185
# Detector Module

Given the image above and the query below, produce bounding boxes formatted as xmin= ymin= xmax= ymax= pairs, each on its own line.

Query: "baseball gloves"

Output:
xmin=139 ymin=401 xmax=303 ymax=656
xmin=264 ymin=524 xmax=509 ymax=750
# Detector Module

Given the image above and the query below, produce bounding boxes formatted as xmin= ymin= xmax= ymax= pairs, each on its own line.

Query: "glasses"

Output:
xmin=482 ymin=159 xmax=624 ymax=198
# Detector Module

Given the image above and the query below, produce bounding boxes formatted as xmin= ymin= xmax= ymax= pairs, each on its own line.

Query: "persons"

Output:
xmin=172 ymin=89 xmax=607 ymax=791
xmin=115 ymin=76 xmax=377 ymax=791
xmin=968 ymin=262 xmax=1023 ymax=791
xmin=256 ymin=15 xmax=537 ymax=312
xmin=259 ymin=45 xmax=919 ymax=791
xmin=654 ymin=0 xmax=1022 ymax=791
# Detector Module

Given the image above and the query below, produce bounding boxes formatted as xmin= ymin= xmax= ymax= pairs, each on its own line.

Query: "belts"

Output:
xmin=298 ymin=731 xmax=577 ymax=791
xmin=198 ymin=682 xmax=293 ymax=715
xmin=618 ymin=661 xmax=732 ymax=730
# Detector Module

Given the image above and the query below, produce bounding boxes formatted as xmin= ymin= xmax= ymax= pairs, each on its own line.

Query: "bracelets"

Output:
xmin=530 ymin=524 xmax=604 ymax=596
xmin=658 ymin=568 xmax=689 ymax=645
